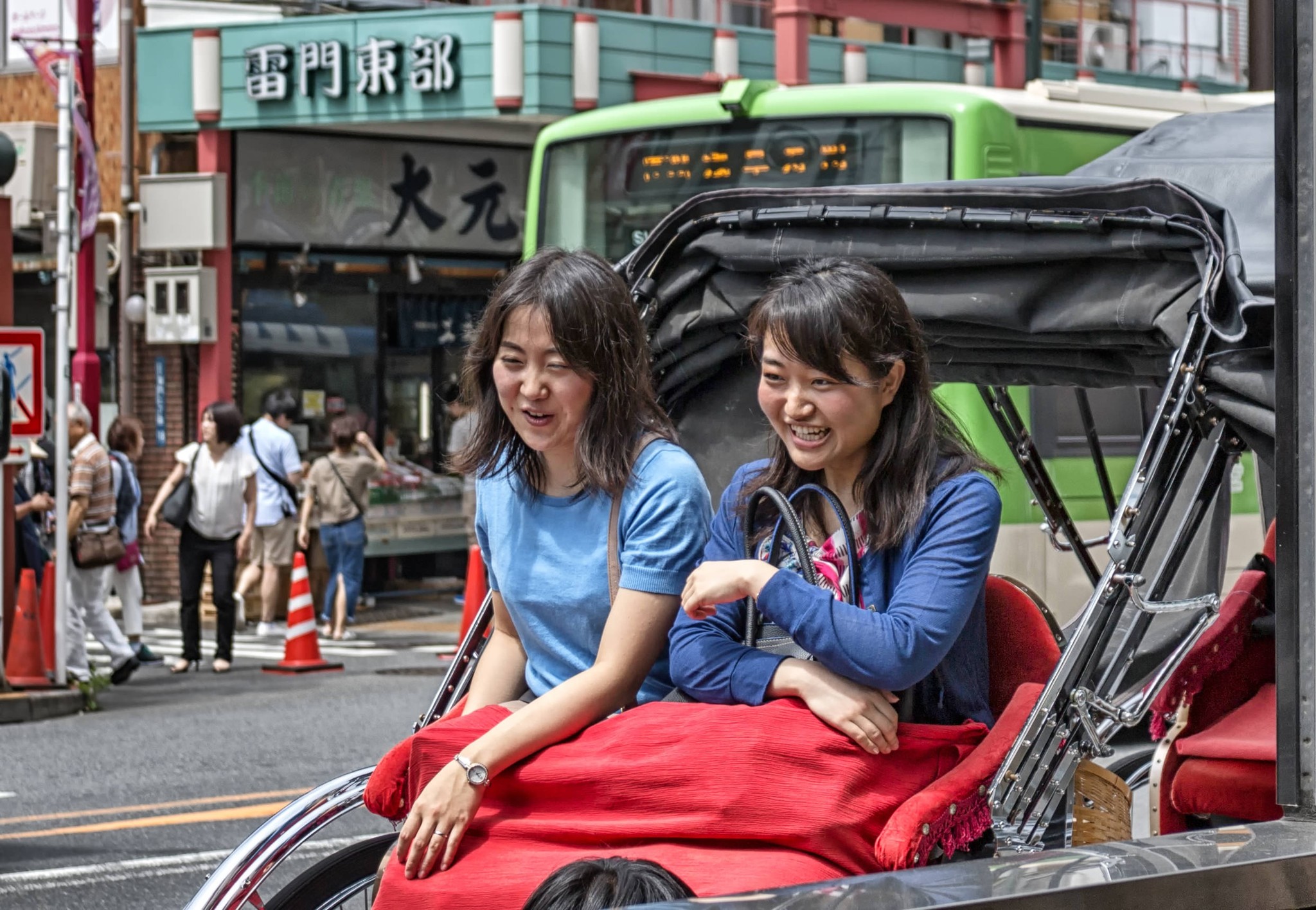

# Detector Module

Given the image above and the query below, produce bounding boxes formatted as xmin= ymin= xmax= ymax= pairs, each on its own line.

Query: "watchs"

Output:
xmin=453 ymin=754 xmax=493 ymax=789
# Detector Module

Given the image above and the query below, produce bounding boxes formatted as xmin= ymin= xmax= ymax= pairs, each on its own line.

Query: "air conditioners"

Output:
xmin=1053 ymin=17 xmax=1127 ymax=73
xmin=0 ymin=121 xmax=59 ymax=228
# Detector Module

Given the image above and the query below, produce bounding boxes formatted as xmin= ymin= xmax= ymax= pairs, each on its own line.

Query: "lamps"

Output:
xmin=407 ymin=253 xmax=423 ymax=284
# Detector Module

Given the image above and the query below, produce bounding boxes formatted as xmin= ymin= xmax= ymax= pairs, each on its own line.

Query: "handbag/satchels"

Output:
xmin=115 ymin=540 xmax=139 ymax=573
xmin=67 ymin=525 xmax=125 ymax=570
xmin=663 ymin=482 xmax=913 ymax=723
xmin=161 ymin=444 xmax=203 ymax=529
xmin=279 ymin=480 xmax=300 ymax=520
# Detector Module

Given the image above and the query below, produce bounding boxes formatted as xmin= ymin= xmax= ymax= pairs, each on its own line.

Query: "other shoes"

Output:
xmin=233 ymin=592 xmax=246 ymax=630
xmin=257 ymin=621 xmax=285 ymax=637
xmin=109 ymin=657 xmax=139 ymax=686
xmin=49 ymin=671 xmax=76 ymax=684
xmin=134 ymin=642 xmax=160 ymax=663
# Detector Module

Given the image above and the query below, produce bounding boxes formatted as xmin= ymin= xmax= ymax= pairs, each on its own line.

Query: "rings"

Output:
xmin=433 ymin=831 xmax=447 ymax=839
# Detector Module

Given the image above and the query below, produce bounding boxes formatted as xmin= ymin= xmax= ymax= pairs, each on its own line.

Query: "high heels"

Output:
xmin=213 ymin=659 xmax=230 ymax=672
xmin=170 ymin=658 xmax=198 ymax=673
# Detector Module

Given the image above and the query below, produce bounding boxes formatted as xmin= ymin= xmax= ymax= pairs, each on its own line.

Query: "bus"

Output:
xmin=518 ymin=79 xmax=1278 ymax=670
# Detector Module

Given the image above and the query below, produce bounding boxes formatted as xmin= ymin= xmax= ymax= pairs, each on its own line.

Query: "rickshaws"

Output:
xmin=174 ymin=104 xmax=1283 ymax=909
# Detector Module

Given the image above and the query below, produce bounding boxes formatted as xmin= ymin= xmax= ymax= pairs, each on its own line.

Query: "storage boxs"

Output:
xmin=367 ymin=497 xmax=466 ymax=542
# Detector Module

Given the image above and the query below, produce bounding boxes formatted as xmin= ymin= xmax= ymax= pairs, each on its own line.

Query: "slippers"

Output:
xmin=318 ymin=625 xmax=332 ymax=638
xmin=337 ymin=631 xmax=355 ymax=641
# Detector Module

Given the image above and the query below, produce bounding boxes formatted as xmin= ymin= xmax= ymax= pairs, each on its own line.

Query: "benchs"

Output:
xmin=1148 ymin=516 xmax=1283 ymax=837
xmin=360 ymin=573 xmax=1066 ymax=868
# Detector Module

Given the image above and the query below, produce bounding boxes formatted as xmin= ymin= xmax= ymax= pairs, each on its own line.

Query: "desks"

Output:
xmin=362 ymin=534 xmax=466 ymax=606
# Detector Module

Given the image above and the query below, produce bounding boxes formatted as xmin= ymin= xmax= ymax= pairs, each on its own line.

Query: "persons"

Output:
xmin=10 ymin=382 xmax=480 ymax=686
xmin=666 ymin=253 xmax=996 ymax=755
xmin=395 ymin=245 xmax=713 ymax=880
xmin=523 ymin=858 xmax=695 ymax=910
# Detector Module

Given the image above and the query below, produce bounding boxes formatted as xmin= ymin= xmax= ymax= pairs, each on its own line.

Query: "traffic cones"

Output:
xmin=5 ymin=568 xmax=69 ymax=689
xmin=39 ymin=560 xmax=56 ymax=671
xmin=263 ymin=548 xmax=346 ymax=676
